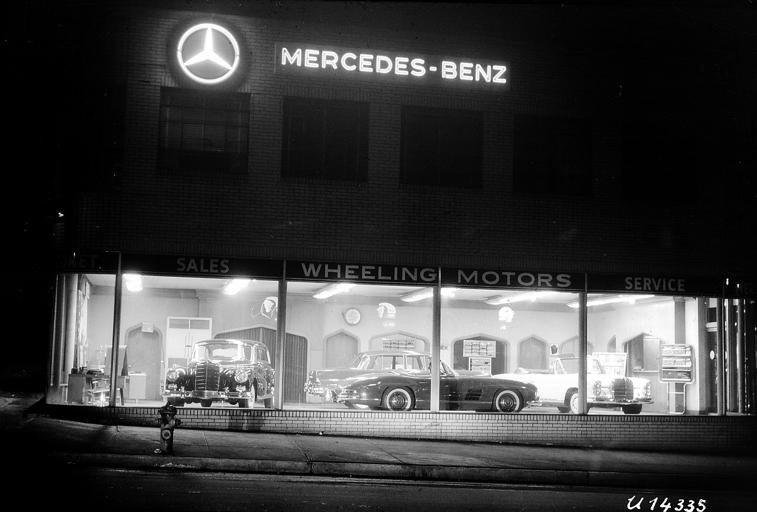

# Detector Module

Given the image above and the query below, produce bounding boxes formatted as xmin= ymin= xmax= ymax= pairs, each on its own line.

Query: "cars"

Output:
xmin=490 ymin=358 xmax=654 ymax=417
xmin=302 ymin=349 xmax=537 ymax=412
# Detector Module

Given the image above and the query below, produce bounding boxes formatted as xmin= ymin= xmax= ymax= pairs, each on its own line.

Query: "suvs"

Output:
xmin=162 ymin=337 xmax=274 ymax=410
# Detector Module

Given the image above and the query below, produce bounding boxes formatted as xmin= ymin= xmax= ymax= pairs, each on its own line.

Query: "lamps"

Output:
xmin=567 ymin=294 xmax=655 ymax=309
xmin=400 ymin=286 xmax=453 ymax=303
xmin=484 ymin=291 xmax=560 ymax=306
xmin=221 ymin=278 xmax=250 ymax=296
xmin=122 ymin=273 xmax=144 ymax=292
xmin=312 ymin=282 xmax=355 ymax=301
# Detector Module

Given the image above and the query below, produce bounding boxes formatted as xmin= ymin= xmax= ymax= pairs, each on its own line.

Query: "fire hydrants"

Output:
xmin=155 ymin=401 xmax=184 ymax=454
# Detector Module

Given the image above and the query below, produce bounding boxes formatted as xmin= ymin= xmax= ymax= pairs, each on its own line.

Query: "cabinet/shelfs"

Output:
xmin=626 ymin=333 xmax=669 ymax=373
xmin=165 ymin=316 xmax=213 ymax=369
xmin=657 ymin=342 xmax=694 ymax=414
xmin=119 ymin=374 xmax=147 ymax=404
